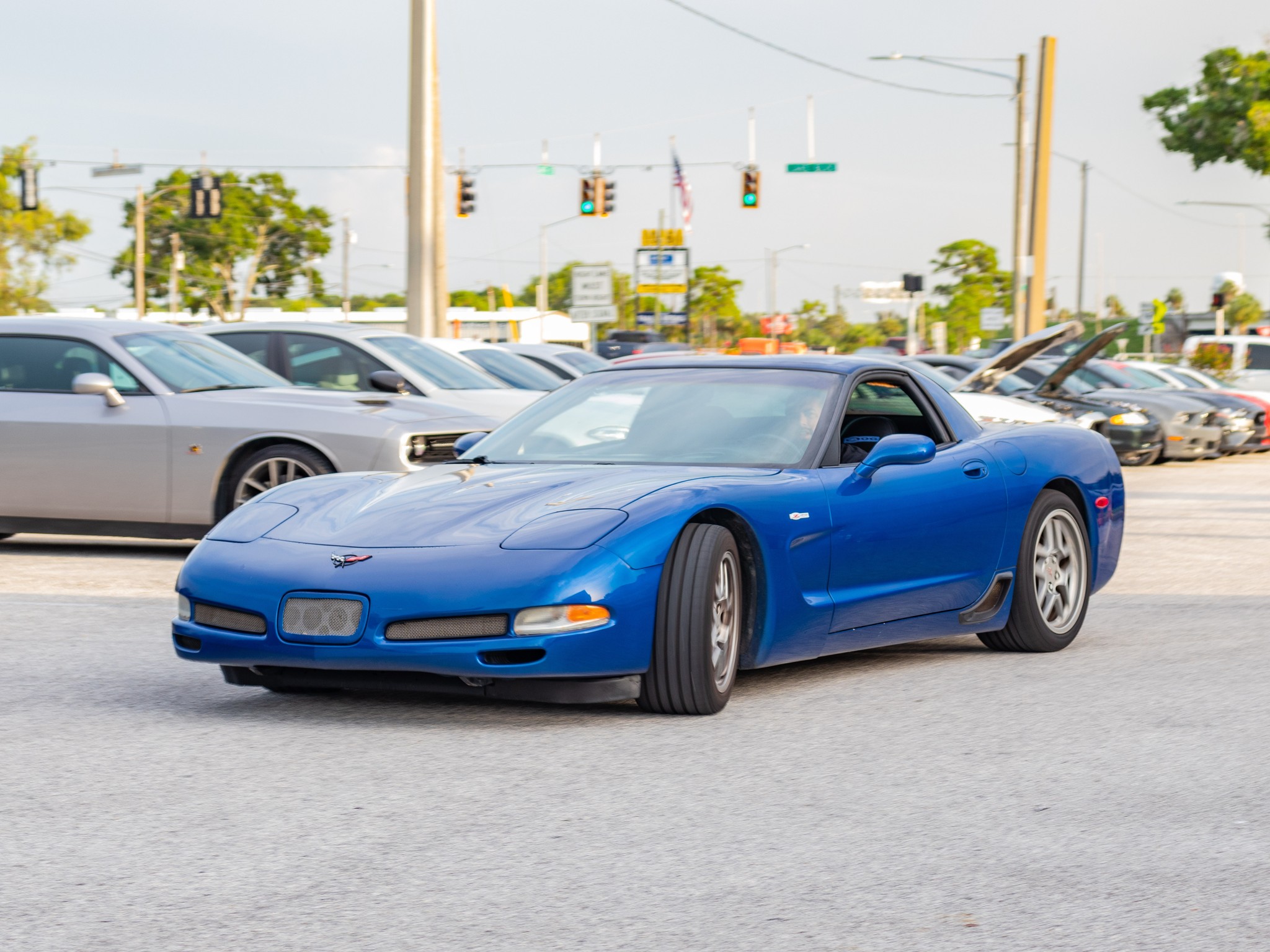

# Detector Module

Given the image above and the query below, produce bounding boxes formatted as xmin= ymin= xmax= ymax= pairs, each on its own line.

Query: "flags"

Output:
xmin=672 ymin=156 xmax=697 ymax=221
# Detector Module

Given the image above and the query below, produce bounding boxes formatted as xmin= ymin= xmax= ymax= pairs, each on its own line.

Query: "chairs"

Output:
xmin=840 ymin=413 xmax=896 ymax=454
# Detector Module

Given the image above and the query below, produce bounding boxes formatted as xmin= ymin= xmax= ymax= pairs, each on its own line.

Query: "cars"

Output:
xmin=169 ymin=356 xmax=1126 ymax=716
xmin=0 ymin=314 xmax=509 ymax=552
xmin=189 ymin=322 xmax=693 ymax=433
xmin=833 ymin=322 xmax=1269 ymax=467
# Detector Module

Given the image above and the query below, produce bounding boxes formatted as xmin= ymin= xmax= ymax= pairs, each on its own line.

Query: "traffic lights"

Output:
xmin=581 ymin=178 xmax=594 ymax=215
xmin=741 ymin=171 xmax=759 ymax=207
xmin=600 ymin=183 xmax=614 ymax=212
xmin=459 ymin=177 xmax=475 ymax=215
